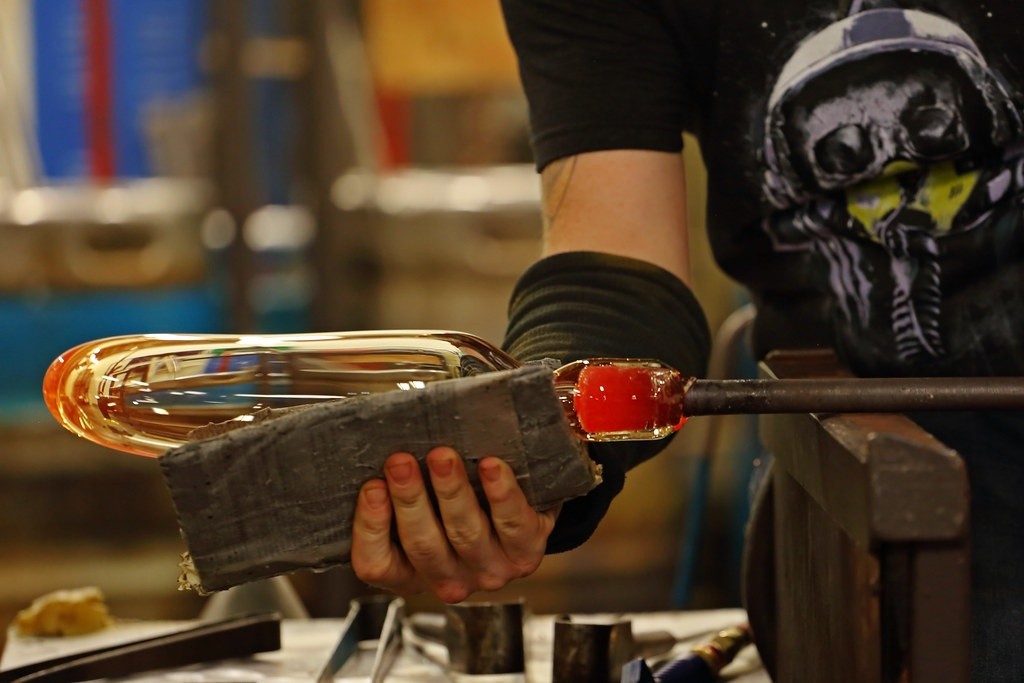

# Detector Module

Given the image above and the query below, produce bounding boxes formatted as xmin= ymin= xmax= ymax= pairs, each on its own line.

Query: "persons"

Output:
xmin=351 ymin=0 xmax=1024 ymax=683
